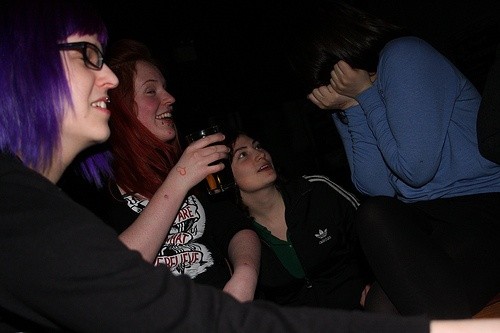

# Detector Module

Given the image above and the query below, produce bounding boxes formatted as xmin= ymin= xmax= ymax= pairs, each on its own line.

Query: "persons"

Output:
xmin=215 ymin=132 xmax=400 ymax=315
xmin=57 ymin=39 xmax=260 ymax=303
xmin=0 ymin=0 xmax=499 ymax=332
xmin=476 ymin=63 xmax=500 ymax=168
xmin=300 ymin=3 xmax=500 ymax=318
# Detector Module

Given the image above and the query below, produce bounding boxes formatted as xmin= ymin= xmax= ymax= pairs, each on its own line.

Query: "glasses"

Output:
xmin=59 ymin=41 xmax=105 ymax=70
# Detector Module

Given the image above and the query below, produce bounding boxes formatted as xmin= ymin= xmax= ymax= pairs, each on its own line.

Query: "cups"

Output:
xmin=187 ymin=126 xmax=237 ymax=196
